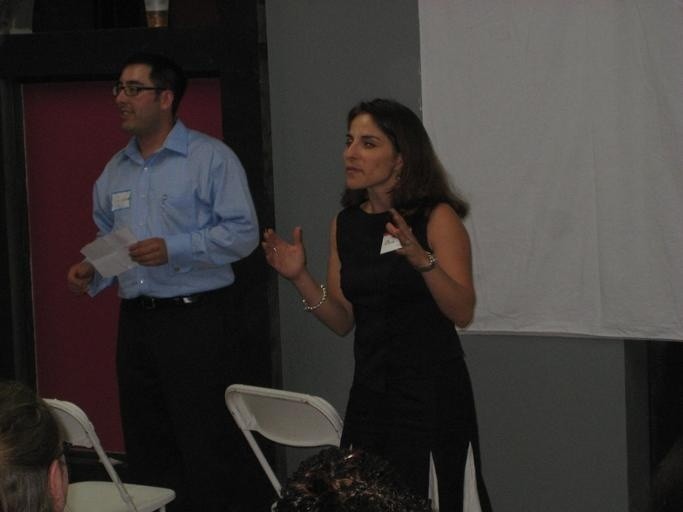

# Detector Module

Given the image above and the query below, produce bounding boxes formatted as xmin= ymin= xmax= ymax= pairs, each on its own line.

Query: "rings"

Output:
xmin=274 ymin=247 xmax=279 ymax=256
xmin=402 ymin=242 xmax=409 ymax=248
xmin=392 ymin=232 xmax=400 ymax=238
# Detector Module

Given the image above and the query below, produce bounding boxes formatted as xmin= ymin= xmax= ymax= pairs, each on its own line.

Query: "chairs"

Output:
xmin=225 ymin=381 xmax=342 ymax=500
xmin=43 ymin=396 xmax=179 ymax=512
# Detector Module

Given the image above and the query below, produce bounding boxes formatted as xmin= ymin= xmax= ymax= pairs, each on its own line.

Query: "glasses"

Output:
xmin=111 ymin=83 xmax=158 ymax=97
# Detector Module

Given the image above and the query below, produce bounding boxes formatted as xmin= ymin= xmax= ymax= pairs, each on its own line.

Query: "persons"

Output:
xmin=271 ymin=447 xmax=434 ymax=512
xmin=262 ymin=97 xmax=491 ymax=512
xmin=0 ymin=382 xmax=69 ymax=512
xmin=66 ymin=54 xmax=260 ymax=512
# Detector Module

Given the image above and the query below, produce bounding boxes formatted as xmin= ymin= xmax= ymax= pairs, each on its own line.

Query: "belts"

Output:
xmin=141 ymin=294 xmax=201 ymax=309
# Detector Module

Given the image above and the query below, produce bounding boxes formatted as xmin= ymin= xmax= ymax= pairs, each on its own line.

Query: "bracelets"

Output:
xmin=301 ymin=284 xmax=327 ymax=311
xmin=421 ymin=250 xmax=436 ymax=271
